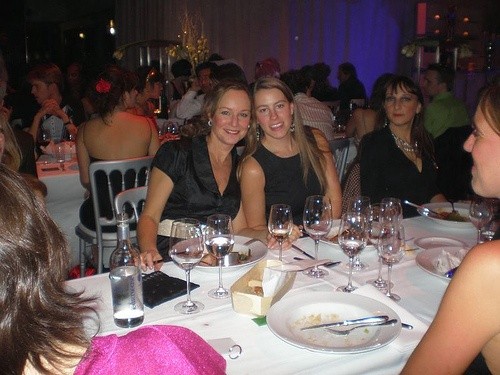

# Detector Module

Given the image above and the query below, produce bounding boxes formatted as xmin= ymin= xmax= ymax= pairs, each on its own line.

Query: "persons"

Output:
xmin=0 ymin=53 xmax=500 ymax=375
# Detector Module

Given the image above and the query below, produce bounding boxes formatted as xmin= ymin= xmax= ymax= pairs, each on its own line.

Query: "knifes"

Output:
xmin=300 ymin=315 xmax=389 ymax=331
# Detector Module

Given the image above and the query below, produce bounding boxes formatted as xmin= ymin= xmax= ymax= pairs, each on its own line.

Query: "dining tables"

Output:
xmin=32 ymin=140 xmax=93 ymax=268
xmin=57 ymin=214 xmax=481 ymax=375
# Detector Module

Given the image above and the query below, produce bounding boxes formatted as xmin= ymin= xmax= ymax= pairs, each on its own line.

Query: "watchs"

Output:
xmin=64 ymin=119 xmax=73 ymax=125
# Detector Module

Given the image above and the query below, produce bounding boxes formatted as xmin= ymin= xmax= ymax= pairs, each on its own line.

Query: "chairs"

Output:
xmin=74 ymin=139 xmax=349 ymax=278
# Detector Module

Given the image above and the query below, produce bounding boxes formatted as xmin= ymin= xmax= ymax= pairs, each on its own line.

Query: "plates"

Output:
xmin=417 ymin=202 xmax=476 ymax=227
xmin=196 ymin=235 xmax=268 ymax=274
xmin=265 ymin=290 xmax=401 ymax=353
xmin=415 ymin=247 xmax=471 ymax=280
xmin=414 ymin=237 xmax=466 ymax=250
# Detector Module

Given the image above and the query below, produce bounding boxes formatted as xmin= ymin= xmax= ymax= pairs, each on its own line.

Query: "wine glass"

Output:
xmin=169 ymin=218 xmax=206 ymax=315
xmin=39 ymin=129 xmax=78 ymax=175
xmin=367 ymin=199 xmax=403 ymax=288
xmin=302 ymin=196 xmax=332 ymax=279
xmin=267 ymin=204 xmax=293 ymax=262
xmin=336 ymin=213 xmax=369 ymax=293
xmin=346 ymin=196 xmax=373 ymax=273
xmin=470 ymin=200 xmax=500 ymax=244
xmin=379 ymin=223 xmax=404 ymax=303
xmin=205 ymin=216 xmax=231 ymax=299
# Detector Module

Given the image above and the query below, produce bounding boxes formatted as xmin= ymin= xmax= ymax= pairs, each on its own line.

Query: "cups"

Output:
xmin=159 ymin=121 xmax=185 ymax=134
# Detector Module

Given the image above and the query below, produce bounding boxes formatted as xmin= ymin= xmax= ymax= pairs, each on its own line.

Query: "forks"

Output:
xmin=328 ymin=319 xmax=396 ymax=336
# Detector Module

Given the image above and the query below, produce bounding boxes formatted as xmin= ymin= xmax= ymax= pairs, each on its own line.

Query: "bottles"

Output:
xmin=109 ymin=213 xmax=144 ymax=328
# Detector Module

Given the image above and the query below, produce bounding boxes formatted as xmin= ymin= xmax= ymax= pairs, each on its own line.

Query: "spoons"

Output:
xmin=292 ymin=245 xmax=341 ymax=266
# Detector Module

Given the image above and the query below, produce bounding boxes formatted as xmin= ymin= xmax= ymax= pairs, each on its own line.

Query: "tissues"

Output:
xmin=230 ymin=256 xmax=335 ymax=316
xmin=36 ymin=140 xmax=64 ymax=177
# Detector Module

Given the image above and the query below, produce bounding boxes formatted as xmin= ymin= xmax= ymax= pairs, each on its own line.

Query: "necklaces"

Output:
xmin=393 ymin=133 xmax=419 ymax=154
